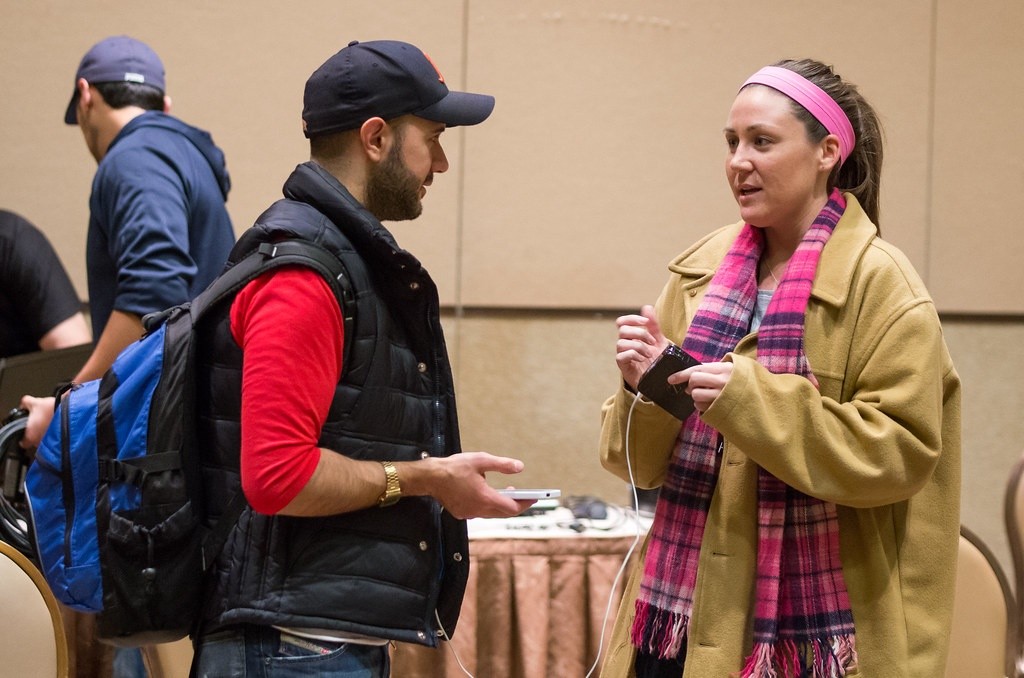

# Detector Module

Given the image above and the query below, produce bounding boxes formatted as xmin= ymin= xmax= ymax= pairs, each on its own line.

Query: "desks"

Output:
xmin=392 ymin=508 xmax=654 ymax=678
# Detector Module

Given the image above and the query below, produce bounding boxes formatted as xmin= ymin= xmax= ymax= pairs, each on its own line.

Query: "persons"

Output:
xmin=178 ymin=39 xmax=537 ymax=678
xmin=597 ymin=55 xmax=959 ymax=678
xmin=14 ymin=33 xmax=235 ymax=678
xmin=0 ymin=210 xmax=93 ymax=354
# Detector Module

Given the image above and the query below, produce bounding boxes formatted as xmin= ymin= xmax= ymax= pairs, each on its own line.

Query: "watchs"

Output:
xmin=373 ymin=458 xmax=402 ymax=507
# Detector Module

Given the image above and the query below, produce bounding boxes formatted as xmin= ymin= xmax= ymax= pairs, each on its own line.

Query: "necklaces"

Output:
xmin=760 ymin=253 xmax=781 ymax=286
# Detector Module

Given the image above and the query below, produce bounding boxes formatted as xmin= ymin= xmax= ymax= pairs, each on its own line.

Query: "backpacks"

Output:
xmin=23 ymin=241 xmax=354 ymax=625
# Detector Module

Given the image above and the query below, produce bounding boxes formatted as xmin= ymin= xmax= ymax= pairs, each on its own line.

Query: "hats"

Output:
xmin=64 ymin=34 xmax=165 ymax=125
xmin=302 ymin=40 xmax=496 ymax=138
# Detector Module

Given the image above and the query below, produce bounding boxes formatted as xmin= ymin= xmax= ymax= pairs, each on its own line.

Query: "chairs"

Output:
xmin=0 ymin=540 xmax=68 ymax=678
xmin=1005 ymin=447 xmax=1024 ymax=678
xmin=946 ymin=523 xmax=1017 ymax=678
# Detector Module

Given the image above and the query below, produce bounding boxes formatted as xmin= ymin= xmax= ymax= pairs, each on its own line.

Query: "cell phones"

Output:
xmin=497 ymin=488 xmax=562 ymax=499
